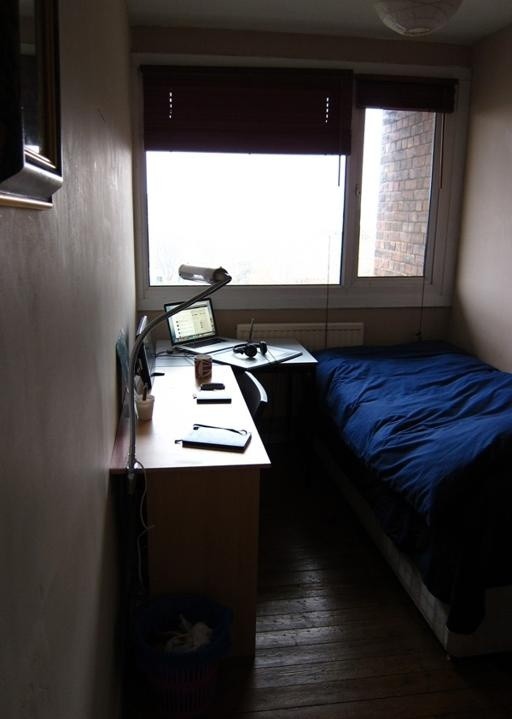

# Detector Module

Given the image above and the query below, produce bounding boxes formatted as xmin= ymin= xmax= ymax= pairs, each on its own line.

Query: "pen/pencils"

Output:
xmin=249 ymin=319 xmax=255 ymax=339
xmin=142 ymin=383 xmax=148 ymax=400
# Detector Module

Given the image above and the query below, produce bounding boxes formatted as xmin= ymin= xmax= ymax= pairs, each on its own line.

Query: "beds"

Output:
xmin=313 ymin=340 xmax=511 ymax=674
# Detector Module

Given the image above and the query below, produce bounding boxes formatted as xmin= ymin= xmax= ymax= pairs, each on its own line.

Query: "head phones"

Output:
xmin=231 ymin=340 xmax=269 ymax=358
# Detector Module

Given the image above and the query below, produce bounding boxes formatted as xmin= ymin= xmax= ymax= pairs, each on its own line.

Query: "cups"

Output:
xmin=194 ymin=354 xmax=212 ymax=378
xmin=135 ymin=395 xmax=154 ymax=421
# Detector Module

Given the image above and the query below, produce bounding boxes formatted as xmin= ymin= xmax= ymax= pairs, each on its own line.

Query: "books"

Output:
xmin=183 ymin=423 xmax=251 ymax=452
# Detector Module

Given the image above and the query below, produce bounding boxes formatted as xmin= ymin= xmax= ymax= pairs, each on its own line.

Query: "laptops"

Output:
xmin=162 ymin=298 xmax=247 ymax=355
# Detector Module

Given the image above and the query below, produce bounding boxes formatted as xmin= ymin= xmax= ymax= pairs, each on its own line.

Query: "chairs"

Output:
xmin=240 ymin=372 xmax=268 ymax=419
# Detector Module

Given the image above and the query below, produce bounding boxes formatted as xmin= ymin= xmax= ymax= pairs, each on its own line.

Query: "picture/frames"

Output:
xmin=0 ymin=0 xmax=63 ymax=210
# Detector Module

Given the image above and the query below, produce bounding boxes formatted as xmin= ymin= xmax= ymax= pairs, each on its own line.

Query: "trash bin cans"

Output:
xmin=122 ymin=594 xmax=229 ymax=719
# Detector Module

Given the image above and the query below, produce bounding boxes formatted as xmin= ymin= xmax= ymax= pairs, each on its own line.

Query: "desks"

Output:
xmin=135 ymin=336 xmax=316 ymax=460
xmin=110 ymin=366 xmax=273 ymax=700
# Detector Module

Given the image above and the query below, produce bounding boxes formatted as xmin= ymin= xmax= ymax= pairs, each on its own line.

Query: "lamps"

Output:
xmin=373 ymin=0 xmax=462 ymax=37
xmin=121 ymin=265 xmax=231 ymax=481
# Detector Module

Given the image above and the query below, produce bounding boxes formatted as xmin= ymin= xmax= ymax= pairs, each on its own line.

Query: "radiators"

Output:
xmin=237 ymin=322 xmax=364 ymax=350
